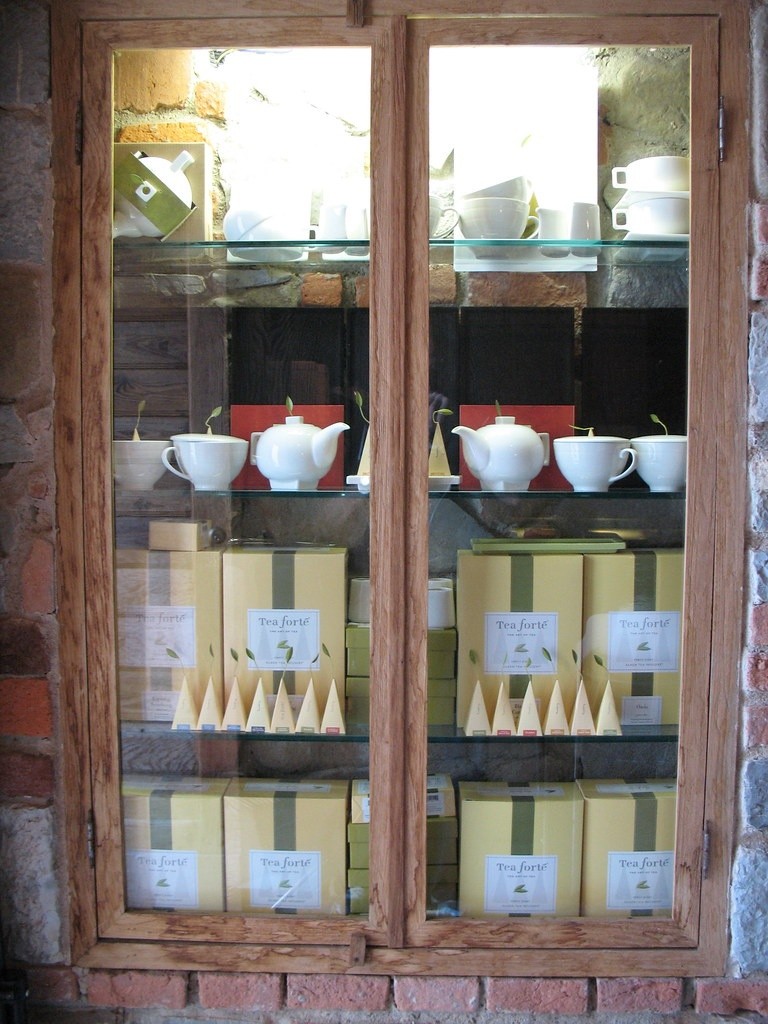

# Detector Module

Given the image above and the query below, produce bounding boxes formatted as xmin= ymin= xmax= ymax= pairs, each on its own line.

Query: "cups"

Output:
xmin=161 ymin=434 xmax=249 ymax=492
xmin=630 ymin=434 xmax=688 ymax=493
xmin=110 ymin=439 xmax=172 ymax=491
xmin=428 ymin=174 xmax=603 ymax=257
xmin=348 ymin=575 xmax=372 ymax=623
xmin=554 ymin=435 xmax=639 ymax=493
xmin=428 ymin=577 xmax=456 ymax=630
xmin=610 ymin=157 xmax=692 ymax=239
xmin=221 ymin=187 xmax=370 ymax=258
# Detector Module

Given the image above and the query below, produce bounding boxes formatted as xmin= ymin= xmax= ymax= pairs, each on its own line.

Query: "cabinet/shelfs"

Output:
xmin=96 ymin=240 xmax=714 ymax=950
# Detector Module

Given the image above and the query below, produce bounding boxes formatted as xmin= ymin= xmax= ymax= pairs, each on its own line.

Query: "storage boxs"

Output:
xmin=105 ymin=536 xmax=685 ymax=918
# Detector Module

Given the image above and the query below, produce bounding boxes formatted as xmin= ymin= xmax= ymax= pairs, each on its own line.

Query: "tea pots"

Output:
xmin=111 ymin=149 xmax=194 ymax=238
xmin=451 ymin=415 xmax=550 ymax=494
xmin=246 ymin=414 xmax=350 ymax=491
